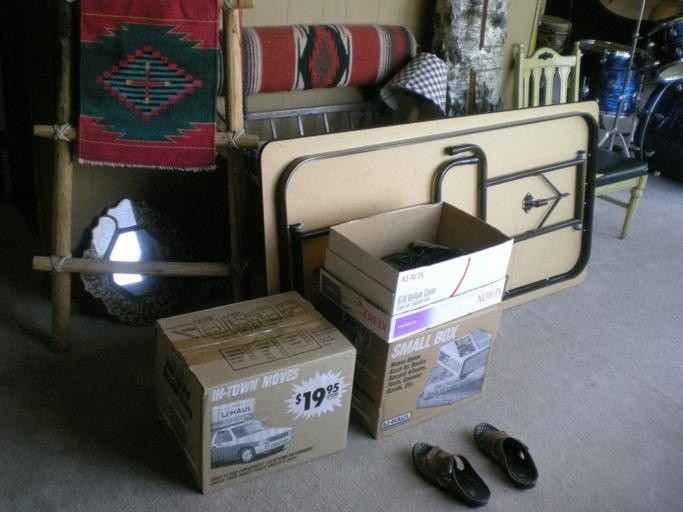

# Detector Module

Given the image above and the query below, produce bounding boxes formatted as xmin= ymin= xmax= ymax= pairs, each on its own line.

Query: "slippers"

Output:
xmin=475 ymin=422 xmax=539 ymax=488
xmin=411 ymin=440 xmax=491 ymax=505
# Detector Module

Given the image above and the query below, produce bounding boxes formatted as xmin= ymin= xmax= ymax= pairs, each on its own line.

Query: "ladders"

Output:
xmin=33 ymin=0 xmax=258 ymax=346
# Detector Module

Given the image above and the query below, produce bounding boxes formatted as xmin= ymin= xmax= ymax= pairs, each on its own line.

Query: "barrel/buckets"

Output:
xmin=537 ymin=14 xmax=573 ymax=58
xmin=377 ymin=50 xmax=450 ymax=115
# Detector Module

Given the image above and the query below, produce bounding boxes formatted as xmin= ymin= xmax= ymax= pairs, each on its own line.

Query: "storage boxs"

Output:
xmin=321 ymin=200 xmax=513 ymax=345
xmin=320 ymin=291 xmax=502 ymax=441
xmin=151 ymin=293 xmax=357 ymax=496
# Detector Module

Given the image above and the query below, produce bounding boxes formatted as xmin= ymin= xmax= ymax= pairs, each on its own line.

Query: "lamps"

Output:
xmin=379 ymin=53 xmax=449 ymax=124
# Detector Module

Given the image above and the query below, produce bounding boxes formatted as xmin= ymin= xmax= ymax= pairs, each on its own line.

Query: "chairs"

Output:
xmin=513 ymin=41 xmax=649 ymax=241
